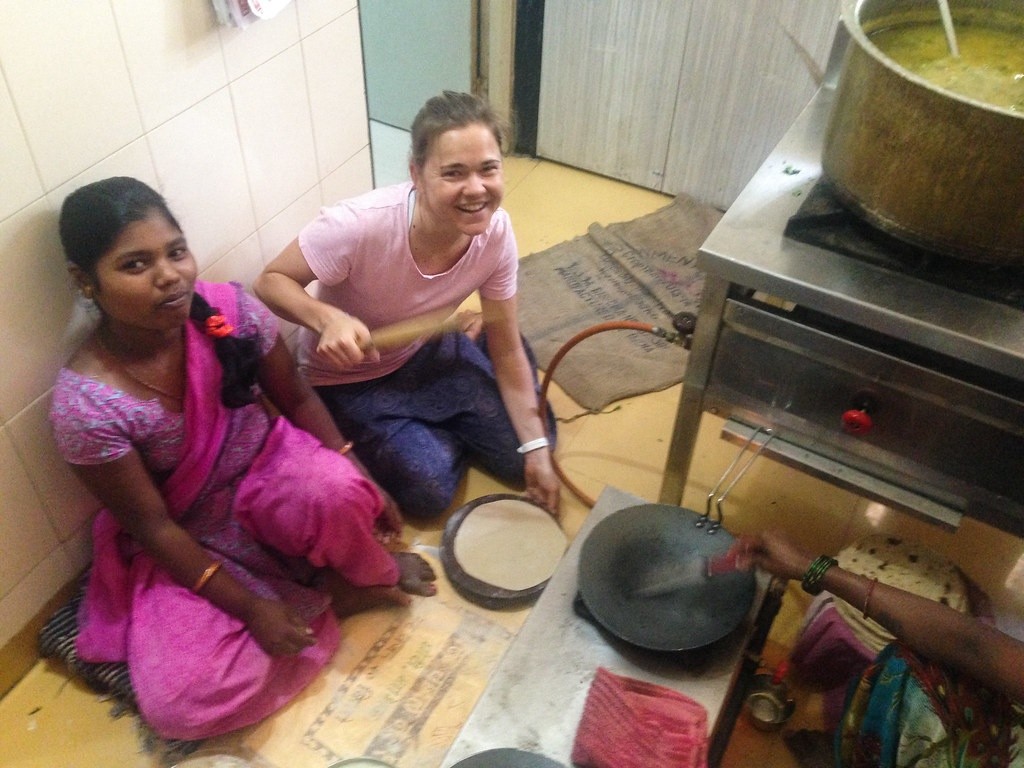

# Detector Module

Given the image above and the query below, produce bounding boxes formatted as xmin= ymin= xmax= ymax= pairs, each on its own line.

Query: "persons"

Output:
xmin=731 ymin=527 xmax=1024 ymax=768
xmin=49 ymin=176 xmax=437 ymax=740
xmin=253 ymin=88 xmax=562 ymax=520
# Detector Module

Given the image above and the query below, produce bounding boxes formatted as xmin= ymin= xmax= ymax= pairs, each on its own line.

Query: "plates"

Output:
xmin=576 ymin=502 xmax=755 ymax=654
xmin=440 ymin=493 xmax=556 ymax=599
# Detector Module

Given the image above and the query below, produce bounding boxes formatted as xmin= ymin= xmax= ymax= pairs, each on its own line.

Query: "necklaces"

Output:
xmin=94 ymin=333 xmax=184 ymax=401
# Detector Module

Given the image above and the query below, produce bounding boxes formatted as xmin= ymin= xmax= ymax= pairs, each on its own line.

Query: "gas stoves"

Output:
xmin=659 ymin=78 xmax=1024 ymax=535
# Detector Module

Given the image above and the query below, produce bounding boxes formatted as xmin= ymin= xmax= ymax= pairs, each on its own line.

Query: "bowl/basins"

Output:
xmin=749 ymin=673 xmax=796 ymax=731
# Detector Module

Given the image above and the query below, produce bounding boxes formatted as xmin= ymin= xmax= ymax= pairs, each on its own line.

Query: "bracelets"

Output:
xmin=517 ymin=437 xmax=550 ymax=454
xmin=803 ymin=554 xmax=838 ymax=595
xmin=863 ymin=577 xmax=878 ymax=618
xmin=193 ymin=560 xmax=222 ymax=593
xmin=338 ymin=440 xmax=354 ymax=454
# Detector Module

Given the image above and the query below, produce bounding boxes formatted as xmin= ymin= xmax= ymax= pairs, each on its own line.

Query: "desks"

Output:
xmin=437 ymin=487 xmax=1024 ymax=767
